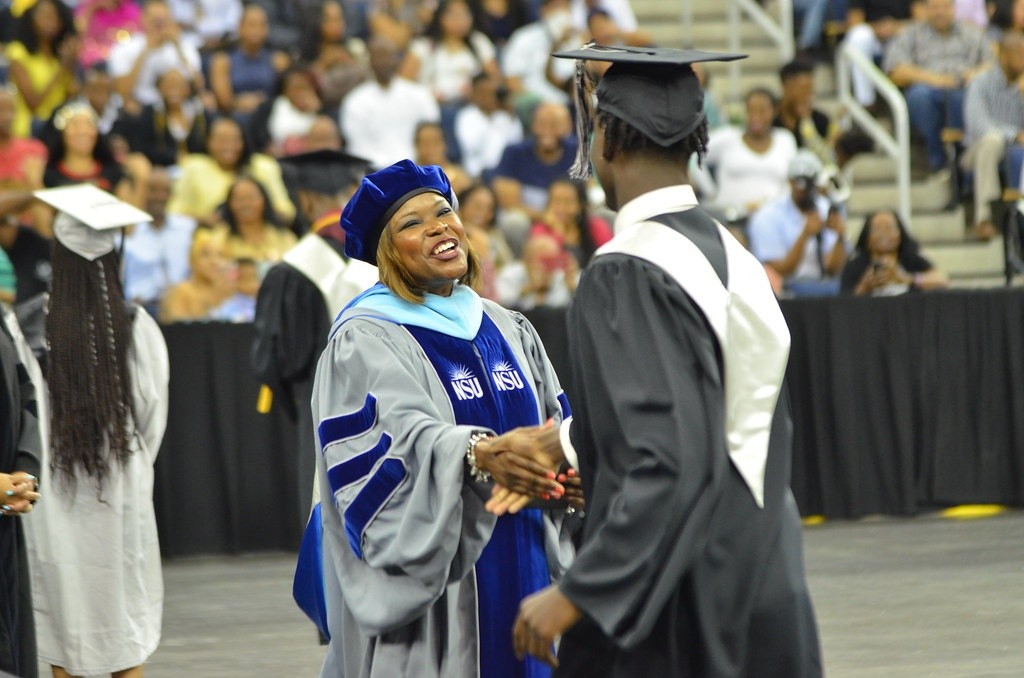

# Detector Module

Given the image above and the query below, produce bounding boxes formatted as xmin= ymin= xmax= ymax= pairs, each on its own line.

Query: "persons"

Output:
xmin=0 ymin=298 xmax=48 ymax=678
xmin=252 ymin=147 xmax=387 ymax=535
xmin=0 ymin=0 xmax=1024 ymax=321
xmin=292 ymin=161 xmax=588 ymax=677
xmin=483 ymin=36 xmax=824 ymax=678
xmin=15 ymin=182 xmax=169 ymax=678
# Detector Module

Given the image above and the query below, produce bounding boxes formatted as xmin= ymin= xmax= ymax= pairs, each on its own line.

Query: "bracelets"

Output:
xmin=466 ymin=433 xmax=492 ymax=484
xmin=566 ymin=505 xmax=577 ymax=518
xmin=915 ymin=272 xmax=922 ymax=288
xmin=27 ymin=475 xmax=39 ymax=488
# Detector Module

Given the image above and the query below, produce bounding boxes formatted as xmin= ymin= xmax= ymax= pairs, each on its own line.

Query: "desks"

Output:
xmin=152 ymin=285 xmax=1024 ymax=565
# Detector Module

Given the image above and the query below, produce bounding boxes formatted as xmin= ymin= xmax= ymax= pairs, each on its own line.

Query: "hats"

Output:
xmin=786 ymin=154 xmax=820 ymax=179
xmin=32 ymin=182 xmax=154 ymax=261
xmin=277 ymin=149 xmax=372 ymax=195
xmin=340 ymin=159 xmax=453 ymax=266
xmin=550 ymin=40 xmax=750 ymax=181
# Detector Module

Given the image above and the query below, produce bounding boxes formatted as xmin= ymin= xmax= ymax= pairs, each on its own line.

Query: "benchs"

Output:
xmin=631 ymin=0 xmax=1024 ymax=287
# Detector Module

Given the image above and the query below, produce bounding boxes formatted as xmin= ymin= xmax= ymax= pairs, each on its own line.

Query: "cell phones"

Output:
xmin=872 ymin=262 xmax=886 ymax=275
